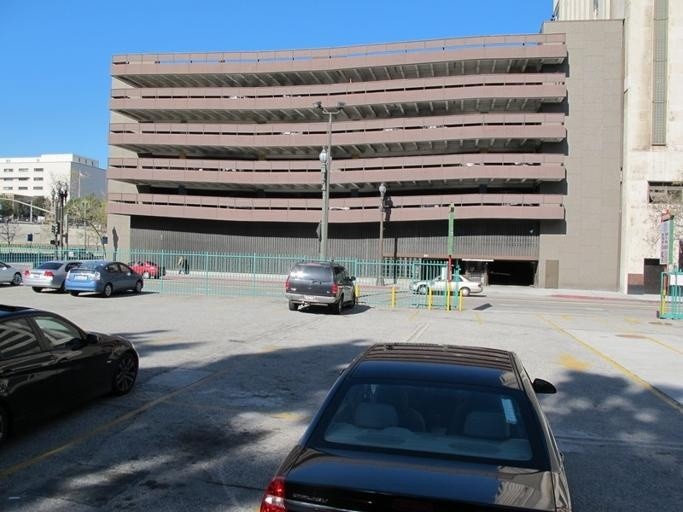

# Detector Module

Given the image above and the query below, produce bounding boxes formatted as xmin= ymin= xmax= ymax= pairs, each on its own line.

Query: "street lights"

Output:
xmin=49 ymin=181 xmax=68 ymax=258
xmin=376 ymin=184 xmax=386 ymax=287
xmin=318 ymin=147 xmax=327 ymax=261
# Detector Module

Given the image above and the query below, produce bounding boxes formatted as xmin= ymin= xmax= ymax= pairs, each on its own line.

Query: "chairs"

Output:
xmin=356 ymin=402 xmax=398 ymax=428
xmin=464 ymin=407 xmax=509 ymax=439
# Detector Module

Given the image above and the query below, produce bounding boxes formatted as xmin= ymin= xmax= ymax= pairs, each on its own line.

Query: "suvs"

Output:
xmin=284 ymin=260 xmax=355 ymax=313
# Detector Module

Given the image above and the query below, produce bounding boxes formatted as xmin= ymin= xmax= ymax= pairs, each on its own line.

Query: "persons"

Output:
xmin=177 ymin=255 xmax=187 ymax=275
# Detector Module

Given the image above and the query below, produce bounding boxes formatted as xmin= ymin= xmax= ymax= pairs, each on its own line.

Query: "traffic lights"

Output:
xmin=51 ymin=221 xmax=59 ymax=236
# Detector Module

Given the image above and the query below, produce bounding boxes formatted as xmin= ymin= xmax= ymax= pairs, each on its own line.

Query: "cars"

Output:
xmin=410 ymin=272 xmax=483 ymax=296
xmin=0 ymin=260 xmax=22 ymax=286
xmin=259 ymin=345 xmax=571 ymax=511
xmin=23 ymin=260 xmax=164 ymax=296
xmin=0 ymin=305 xmax=139 ymax=443
